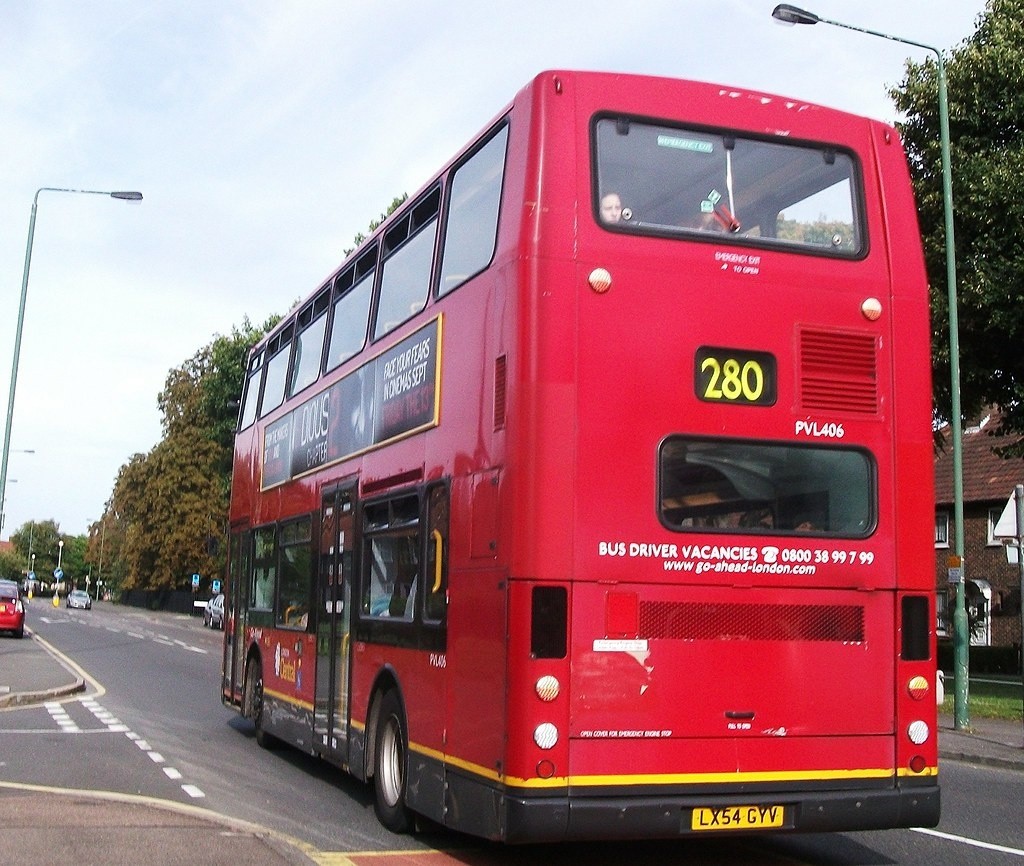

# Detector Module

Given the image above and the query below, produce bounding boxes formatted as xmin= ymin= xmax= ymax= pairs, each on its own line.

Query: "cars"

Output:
xmin=0 ymin=580 xmax=25 ymax=639
xmin=204 ymin=597 xmax=214 ymax=628
xmin=67 ymin=590 xmax=92 ymax=610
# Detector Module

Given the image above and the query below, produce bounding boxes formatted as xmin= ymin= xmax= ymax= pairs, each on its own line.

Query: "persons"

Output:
xmin=256 ymin=568 xmax=276 ymax=608
xmin=670 ymin=464 xmax=818 ymax=532
xmin=601 ymin=192 xmax=622 ymax=225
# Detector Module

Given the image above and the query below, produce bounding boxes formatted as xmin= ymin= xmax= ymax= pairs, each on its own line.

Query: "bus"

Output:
xmin=210 ymin=593 xmax=225 ymax=631
xmin=219 ymin=66 xmax=945 ymax=849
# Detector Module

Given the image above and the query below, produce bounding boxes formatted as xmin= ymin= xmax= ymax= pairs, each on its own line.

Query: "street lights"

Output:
xmin=768 ymin=2 xmax=972 ymax=732
xmin=0 ymin=186 xmax=144 ymax=539
